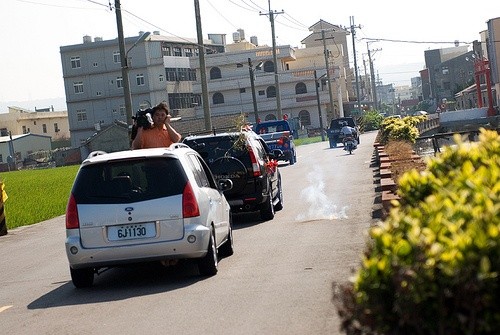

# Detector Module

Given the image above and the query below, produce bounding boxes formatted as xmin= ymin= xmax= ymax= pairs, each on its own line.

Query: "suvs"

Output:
xmin=182 ymin=125 xmax=284 ymax=220
xmin=65 ymin=143 xmax=234 ymax=288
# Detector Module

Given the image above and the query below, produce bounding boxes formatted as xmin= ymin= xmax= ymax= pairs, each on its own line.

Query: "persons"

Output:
xmin=340 ymin=121 xmax=358 ymax=150
xmin=132 ymin=102 xmax=181 ymax=148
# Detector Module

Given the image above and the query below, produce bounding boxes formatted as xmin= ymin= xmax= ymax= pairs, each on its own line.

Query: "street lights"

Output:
xmin=314 ymin=73 xmax=327 ymax=141
xmin=117 ymin=30 xmax=150 ymax=148
xmin=248 ymin=62 xmax=264 ymax=126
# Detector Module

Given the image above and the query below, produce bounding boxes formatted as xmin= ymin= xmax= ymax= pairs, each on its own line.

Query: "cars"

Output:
xmin=413 ymin=111 xmax=428 ymax=116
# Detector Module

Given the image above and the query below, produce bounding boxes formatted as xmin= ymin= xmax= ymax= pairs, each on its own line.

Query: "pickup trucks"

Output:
xmin=326 ymin=117 xmax=360 ymax=148
xmin=253 ymin=121 xmax=296 ymax=165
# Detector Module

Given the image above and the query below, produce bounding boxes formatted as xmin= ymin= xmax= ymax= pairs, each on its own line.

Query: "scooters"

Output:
xmin=340 ymin=131 xmax=358 ymax=154
